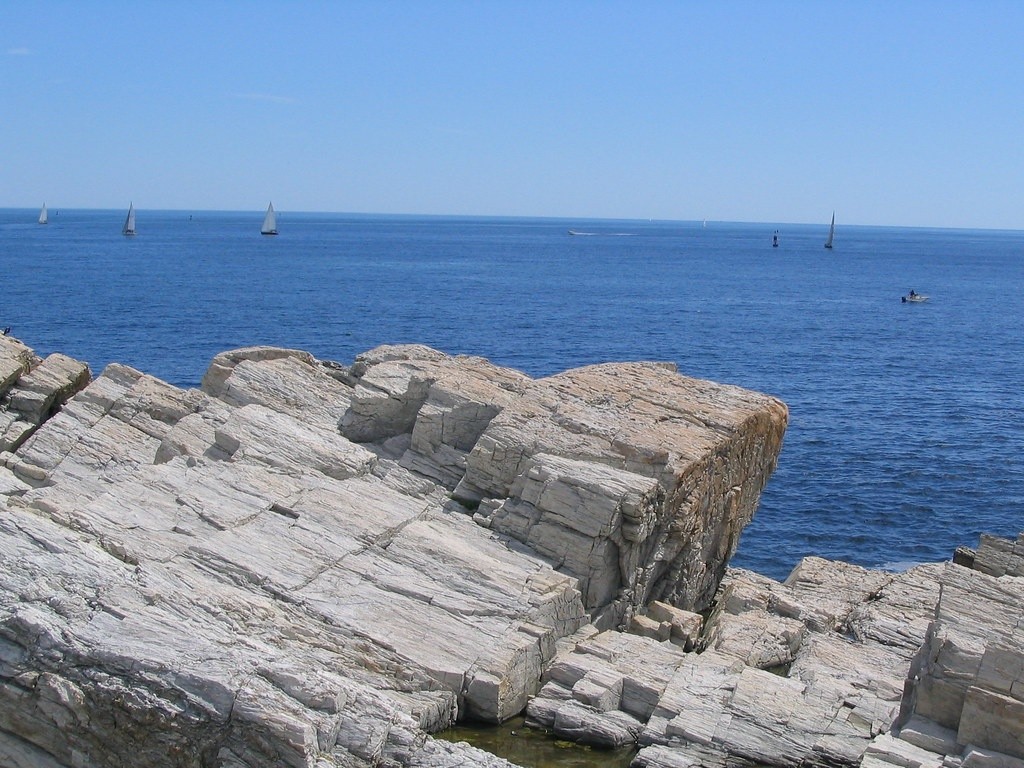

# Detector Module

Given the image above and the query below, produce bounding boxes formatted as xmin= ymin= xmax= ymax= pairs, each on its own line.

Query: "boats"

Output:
xmin=902 ymin=290 xmax=930 ymax=303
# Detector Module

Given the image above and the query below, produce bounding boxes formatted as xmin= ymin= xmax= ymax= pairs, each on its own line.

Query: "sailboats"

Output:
xmin=773 ymin=230 xmax=778 ymax=247
xmin=38 ymin=201 xmax=48 ymax=224
xmin=260 ymin=201 xmax=278 ymax=235
xmin=825 ymin=211 xmax=835 ymax=249
xmin=121 ymin=201 xmax=137 ymax=235
